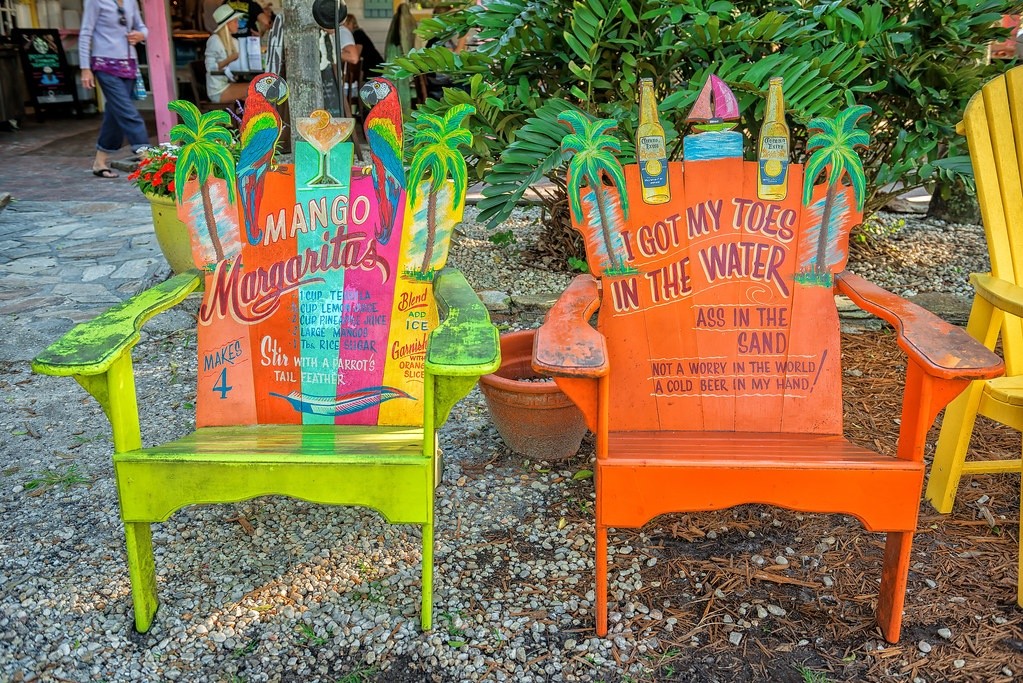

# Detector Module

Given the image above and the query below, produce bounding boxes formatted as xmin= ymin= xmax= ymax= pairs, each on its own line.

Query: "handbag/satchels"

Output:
xmin=131 ymin=68 xmax=148 ymax=102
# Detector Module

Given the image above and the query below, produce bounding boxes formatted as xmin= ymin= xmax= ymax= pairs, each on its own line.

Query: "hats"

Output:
xmin=212 ymin=3 xmax=244 ymax=34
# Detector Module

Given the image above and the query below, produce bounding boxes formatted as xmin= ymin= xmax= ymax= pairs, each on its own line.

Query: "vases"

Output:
xmin=143 ymin=191 xmax=194 ymax=275
xmin=480 ymin=330 xmax=588 ymax=459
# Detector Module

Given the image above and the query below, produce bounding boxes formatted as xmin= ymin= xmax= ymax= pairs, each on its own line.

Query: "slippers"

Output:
xmin=92 ymin=168 xmax=120 ymax=178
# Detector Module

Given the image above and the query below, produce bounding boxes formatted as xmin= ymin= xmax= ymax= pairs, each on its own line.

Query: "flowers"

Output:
xmin=126 ymin=127 xmax=288 ymax=202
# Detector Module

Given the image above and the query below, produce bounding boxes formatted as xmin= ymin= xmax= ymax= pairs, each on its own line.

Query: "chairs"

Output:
xmin=413 ymin=71 xmax=429 ymax=108
xmin=32 ymin=77 xmax=504 ymax=635
xmin=533 ymin=78 xmax=1008 ymax=645
xmin=343 ymin=57 xmax=368 ymax=140
xmin=9 ymin=27 xmax=101 ymax=123
xmin=927 ymin=70 xmax=1022 ymax=605
xmin=188 ymin=62 xmax=236 ymax=127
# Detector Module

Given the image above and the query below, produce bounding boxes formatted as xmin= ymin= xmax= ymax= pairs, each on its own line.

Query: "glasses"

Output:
xmin=117 ymin=6 xmax=127 ymax=27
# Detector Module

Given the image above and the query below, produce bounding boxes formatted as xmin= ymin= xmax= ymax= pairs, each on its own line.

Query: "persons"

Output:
xmin=205 ymin=5 xmax=250 ymax=103
xmin=330 ymin=14 xmax=386 ymax=80
xmin=222 ymin=0 xmax=279 ymax=37
xmin=79 ymin=0 xmax=153 ymax=178
xmin=426 ymin=6 xmax=469 ymax=99
